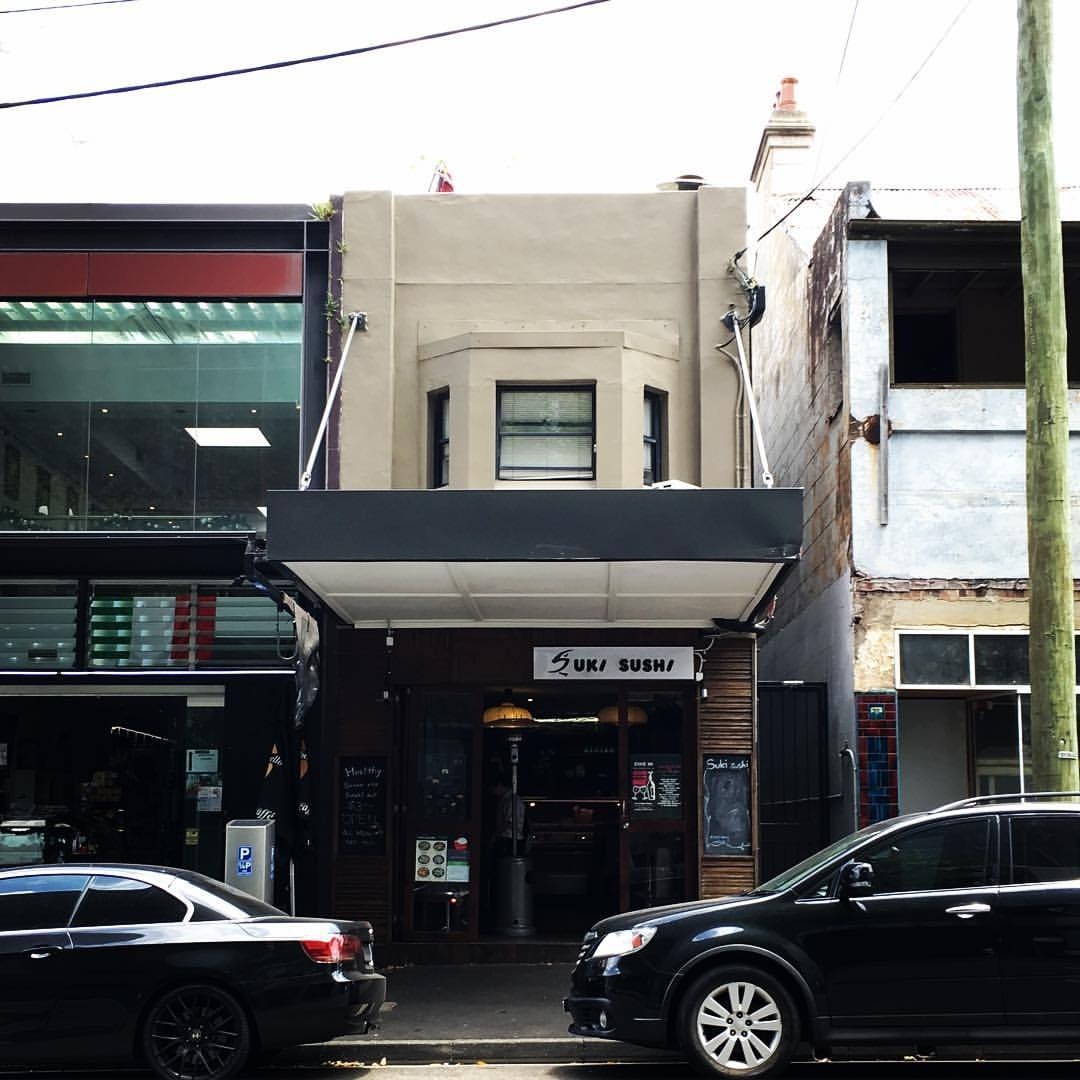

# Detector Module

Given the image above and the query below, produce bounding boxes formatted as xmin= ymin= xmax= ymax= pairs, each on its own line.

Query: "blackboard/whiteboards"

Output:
xmin=700 ymin=748 xmax=756 ymax=861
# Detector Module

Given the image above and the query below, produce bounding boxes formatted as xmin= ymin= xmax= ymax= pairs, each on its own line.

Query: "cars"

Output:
xmin=0 ymin=862 xmax=389 ymax=1079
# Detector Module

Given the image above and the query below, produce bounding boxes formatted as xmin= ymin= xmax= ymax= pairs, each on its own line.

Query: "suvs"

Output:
xmin=562 ymin=790 xmax=1080 ymax=1079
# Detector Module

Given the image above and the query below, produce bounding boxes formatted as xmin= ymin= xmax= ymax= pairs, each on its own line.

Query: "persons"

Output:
xmin=487 ymin=776 xmax=527 ymax=866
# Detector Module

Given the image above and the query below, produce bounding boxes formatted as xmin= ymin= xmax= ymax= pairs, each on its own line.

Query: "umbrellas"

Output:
xmin=254 ymin=675 xmax=327 ymax=918
